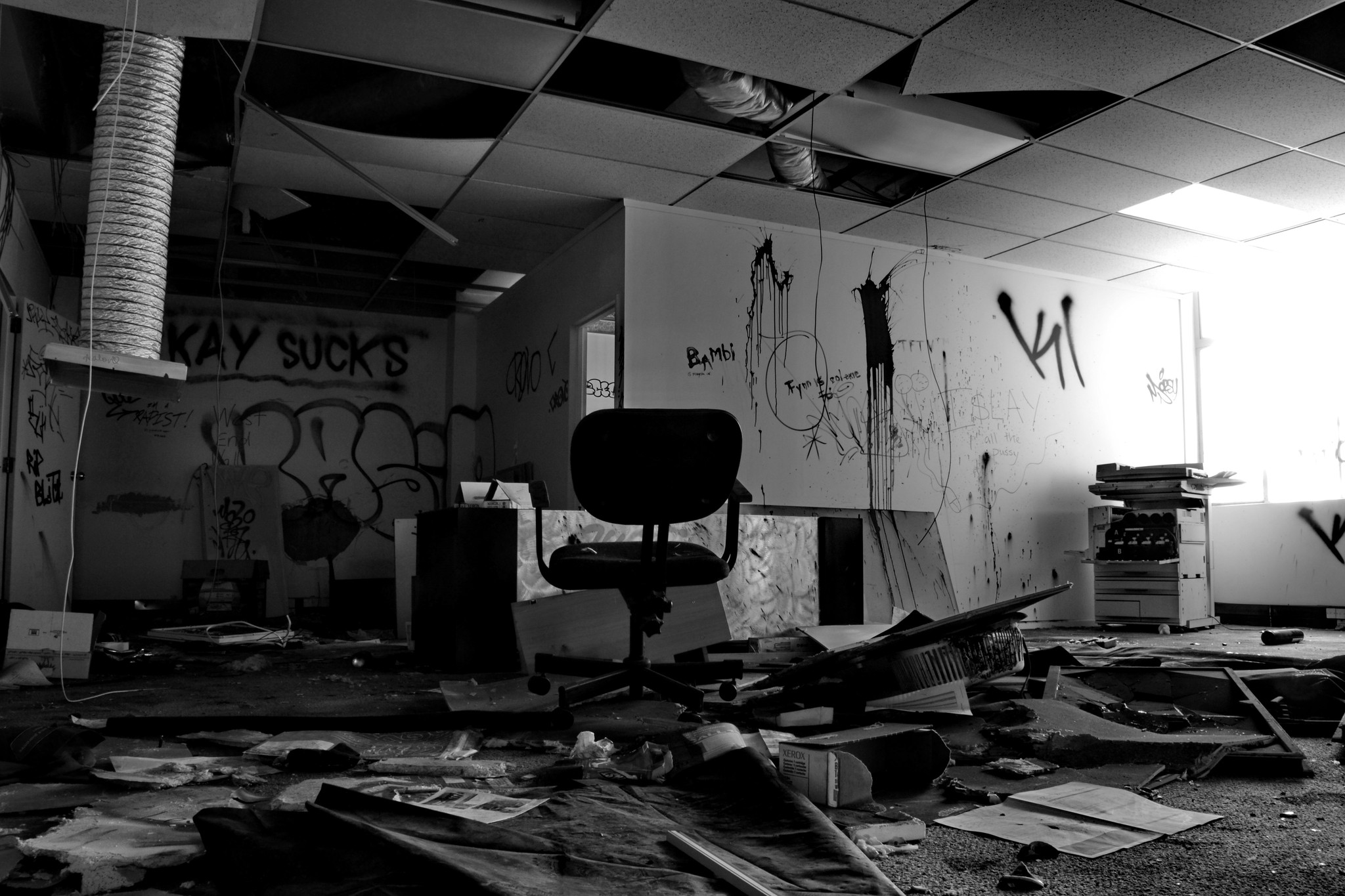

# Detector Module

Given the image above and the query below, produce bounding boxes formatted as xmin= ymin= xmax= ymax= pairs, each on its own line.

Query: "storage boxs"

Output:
xmin=776 ymin=722 xmax=954 ymax=809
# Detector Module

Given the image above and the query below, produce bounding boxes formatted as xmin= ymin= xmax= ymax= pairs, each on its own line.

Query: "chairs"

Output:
xmin=524 ymin=409 xmax=757 ymax=732
